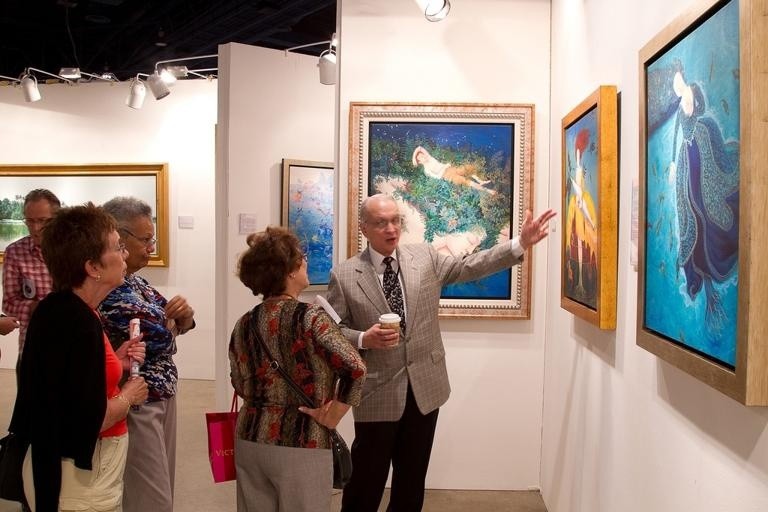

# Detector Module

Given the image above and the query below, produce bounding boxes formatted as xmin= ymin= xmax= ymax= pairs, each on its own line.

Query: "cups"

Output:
xmin=380 ymin=313 xmax=399 ymax=349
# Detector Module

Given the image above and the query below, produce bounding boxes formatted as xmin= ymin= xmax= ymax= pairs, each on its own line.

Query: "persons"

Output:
xmin=412 ymin=143 xmax=496 ymax=195
xmin=9 ymin=202 xmax=149 ymax=510
xmin=563 ymin=131 xmax=597 ymax=289
xmin=0 ymin=316 xmax=24 ymax=336
xmin=665 ymin=61 xmax=737 ymax=303
xmin=2 ymin=187 xmax=62 ymax=368
xmin=323 ymin=192 xmax=559 ymax=511
xmin=223 ymin=227 xmax=368 ymax=509
xmin=96 ymin=199 xmax=197 ymax=509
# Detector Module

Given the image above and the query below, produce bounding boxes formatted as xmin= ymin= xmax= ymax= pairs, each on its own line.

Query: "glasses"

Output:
xmin=101 ymin=243 xmax=126 ymax=255
xmin=22 ymin=217 xmax=50 ymax=226
xmin=365 ymin=217 xmax=403 ymax=227
xmin=121 ymin=227 xmax=159 ymax=250
xmin=295 ymin=253 xmax=308 ymax=262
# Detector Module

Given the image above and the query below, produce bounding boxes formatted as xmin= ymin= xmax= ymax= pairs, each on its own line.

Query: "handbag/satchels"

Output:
xmin=0 ymin=430 xmax=30 ymax=504
xmin=206 ymin=391 xmax=242 ymax=485
xmin=248 ymin=312 xmax=354 ymax=491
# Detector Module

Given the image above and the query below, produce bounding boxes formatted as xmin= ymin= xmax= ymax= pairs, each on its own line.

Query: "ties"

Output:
xmin=382 ymin=256 xmax=407 ymax=336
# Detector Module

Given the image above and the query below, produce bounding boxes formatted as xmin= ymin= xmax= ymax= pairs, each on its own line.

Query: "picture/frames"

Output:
xmin=347 ymin=100 xmax=534 ymax=321
xmin=637 ymin=0 xmax=767 ymax=407
xmin=279 ymin=158 xmax=333 ymax=292
xmin=0 ymin=162 xmax=170 ymax=270
xmin=560 ymin=84 xmax=618 ymax=332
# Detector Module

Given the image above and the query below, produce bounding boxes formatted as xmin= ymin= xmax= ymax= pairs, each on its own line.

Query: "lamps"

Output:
xmin=58 ymin=67 xmax=83 ymax=82
xmin=160 ymin=67 xmax=181 ymax=88
xmin=146 ymin=70 xmax=170 ymax=100
xmin=124 ymin=73 xmax=145 ymax=111
xmin=317 ymin=33 xmax=337 ymax=87
xmin=22 ymin=67 xmax=42 ymax=103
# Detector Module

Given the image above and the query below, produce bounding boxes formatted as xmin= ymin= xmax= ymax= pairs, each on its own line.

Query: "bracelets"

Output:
xmin=112 ymin=393 xmax=135 ymax=420
